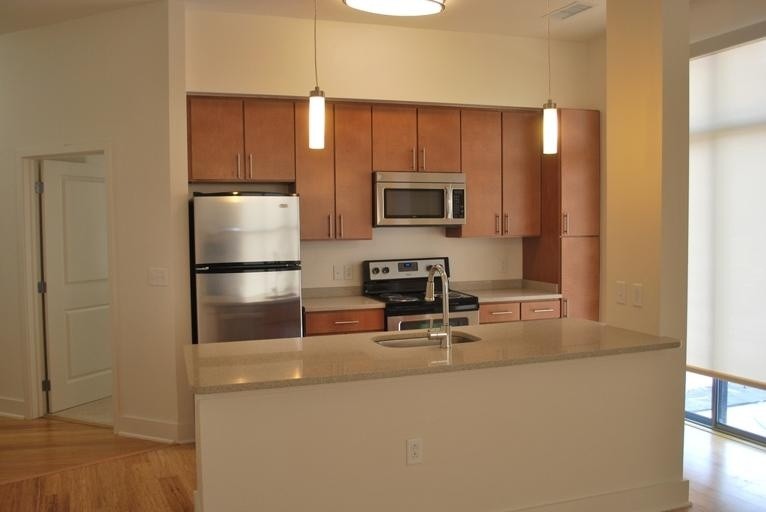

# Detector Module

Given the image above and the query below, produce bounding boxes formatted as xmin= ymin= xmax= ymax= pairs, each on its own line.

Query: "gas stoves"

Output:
xmin=365 ymin=287 xmax=479 ymax=307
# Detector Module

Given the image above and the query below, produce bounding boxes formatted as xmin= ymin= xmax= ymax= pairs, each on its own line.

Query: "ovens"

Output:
xmin=385 ymin=304 xmax=480 ymax=330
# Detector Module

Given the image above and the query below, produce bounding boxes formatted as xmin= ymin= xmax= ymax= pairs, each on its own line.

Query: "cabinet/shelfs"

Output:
xmin=522 ymin=109 xmax=600 ymax=320
xmin=187 ymin=96 xmax=295 ymax=184
xmin=305 ymin=308 xmax=385 ymax=336
xmin=479 ymin=303 xmax=521 ymax=324
xmin=372 ymin=104 xmax=462 ymax=174
xmin=446 ymin=108 xmax=540 ymax=237
xmin=289 ymin=101 xmax=372 ymax=240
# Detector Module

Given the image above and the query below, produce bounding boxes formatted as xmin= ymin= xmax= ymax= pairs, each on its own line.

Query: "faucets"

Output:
xmin=425 ymin=263 xmax=450 ymax=350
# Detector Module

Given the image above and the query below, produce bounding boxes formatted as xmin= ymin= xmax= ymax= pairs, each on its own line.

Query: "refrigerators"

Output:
xmin=191 ymin=190 xmax=304 ymax=344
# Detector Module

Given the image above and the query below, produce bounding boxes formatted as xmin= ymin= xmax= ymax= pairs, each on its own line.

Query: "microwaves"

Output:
xmin=372 ymin=169 xmax=468 ymax=229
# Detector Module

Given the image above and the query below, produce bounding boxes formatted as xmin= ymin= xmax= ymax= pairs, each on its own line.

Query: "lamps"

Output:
xmin=343 ymin=0 xmax=446 ymax=17
xmin=308 ymin=0 xmax=326 ymax=150
xmin=542 ymin=0 xmax=558 ymax=154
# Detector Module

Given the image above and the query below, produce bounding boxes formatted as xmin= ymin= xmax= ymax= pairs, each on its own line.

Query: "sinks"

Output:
xmin=377 ymin=336 xmax=474 ymax=347
